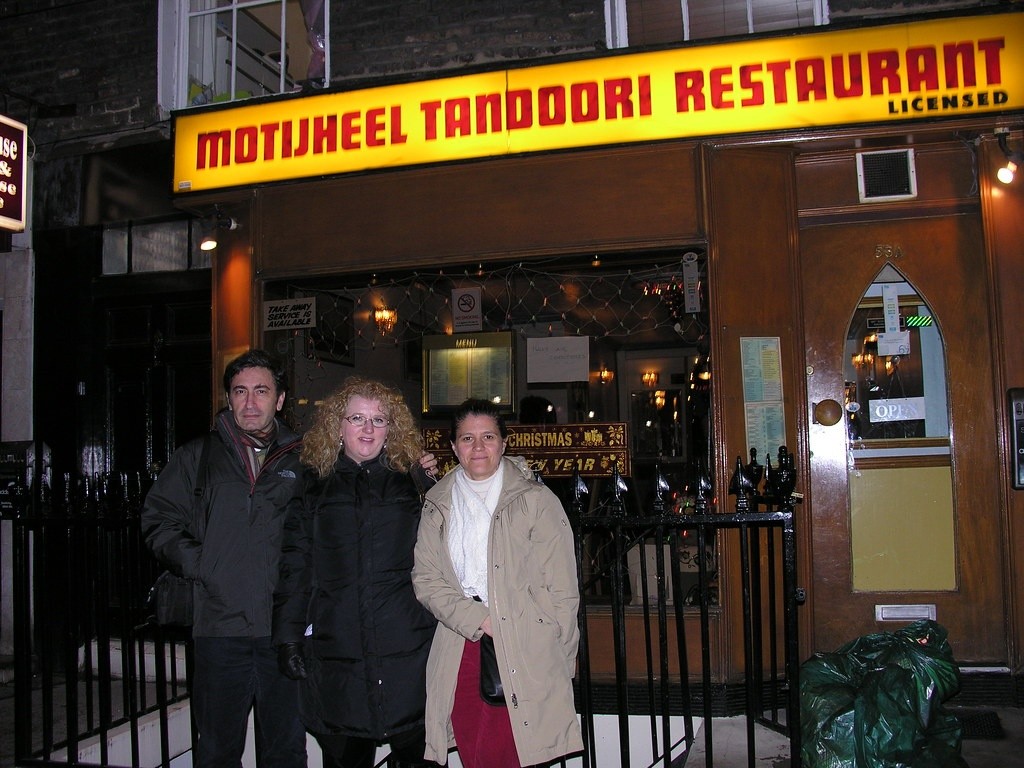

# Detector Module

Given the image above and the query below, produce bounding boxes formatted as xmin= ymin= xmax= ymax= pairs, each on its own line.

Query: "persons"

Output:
xmin=140 ymin=349 xmax=438 ymax=768
xmin=270 ymin=377 xmax=451 ymax=768
xmin=412 ymin=397 xmax=585 ymax=768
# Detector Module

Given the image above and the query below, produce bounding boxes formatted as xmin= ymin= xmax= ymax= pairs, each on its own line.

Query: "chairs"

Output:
xmin=625 ymin=542 xmax=678 ymax=606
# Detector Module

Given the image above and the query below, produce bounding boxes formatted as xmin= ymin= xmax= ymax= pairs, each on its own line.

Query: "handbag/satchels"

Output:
xmin=480 ymin=633 xmax=507 ymax=705
xmin=147 ymin=572 xmax=194 ymax=628
xmin=795 ymin=618 xmax=964 ymax=768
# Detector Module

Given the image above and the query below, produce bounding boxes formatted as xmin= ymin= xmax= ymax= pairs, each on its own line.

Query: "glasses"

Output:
xmin=342 ymin=415 xmax=394 ymax=428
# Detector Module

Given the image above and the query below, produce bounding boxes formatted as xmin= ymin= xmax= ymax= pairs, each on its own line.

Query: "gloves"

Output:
xmin=277 ymin=642 xmax=308 ymax=680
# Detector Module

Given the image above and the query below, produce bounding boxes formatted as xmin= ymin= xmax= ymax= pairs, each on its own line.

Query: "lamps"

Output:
xmin=373 ymin=305 xmax=399 ymax=336
xmin=992 ymin=127 xmax=1024 ymax=184
xmin=641 ymin=372 xmax=659 ymax=389
xmin=194 ymin=216 xmax=240 ymax=252
xmin=655 ymin=390 xmax=667 ymax=408
xmin=850 ymin=328 xmax=899 ymax=377
xmin=597 ymin=367 xmax=610 ymax=385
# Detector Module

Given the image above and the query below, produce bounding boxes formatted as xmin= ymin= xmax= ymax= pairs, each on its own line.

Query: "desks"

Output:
xmin=679 ymin=543 xmax=714 ymax=574
